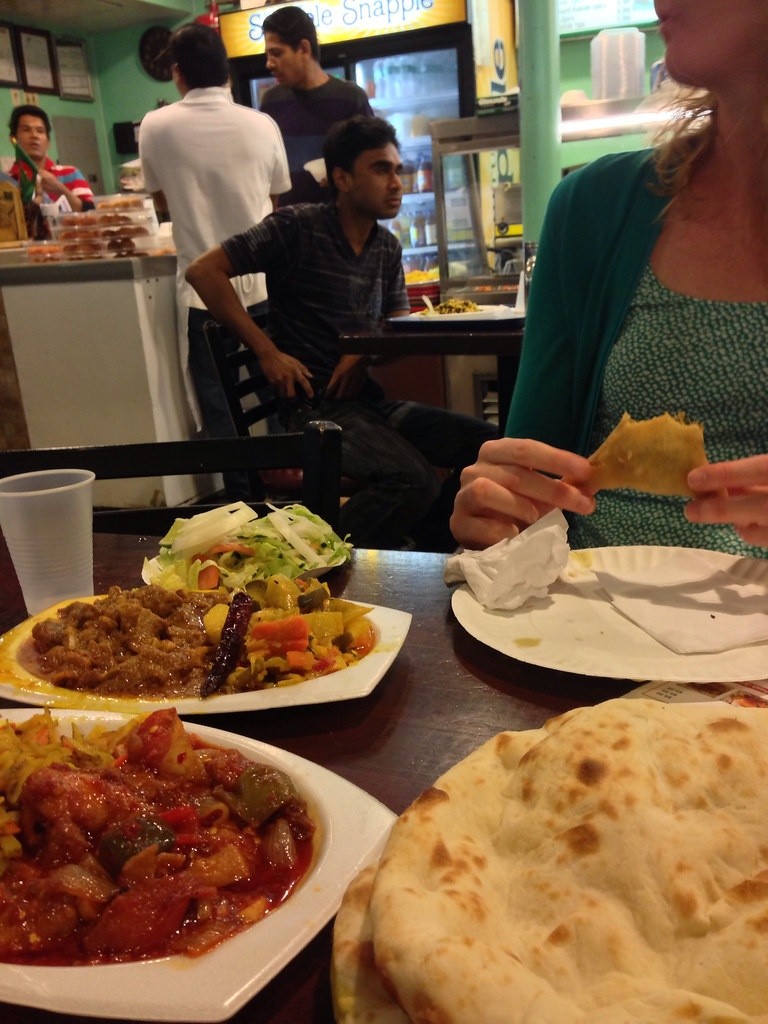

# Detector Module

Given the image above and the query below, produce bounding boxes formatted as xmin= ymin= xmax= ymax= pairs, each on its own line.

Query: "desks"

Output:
xmin=0 ymin=528 xmax=633 ymax=1021
xmin=336 ymin=304 xmax=523 ymax=440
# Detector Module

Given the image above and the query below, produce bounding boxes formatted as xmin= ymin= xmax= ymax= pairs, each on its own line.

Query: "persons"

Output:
xmin=7 ymin=104 xmax=95 ymax=240
xmin=185 ymin=116 xmax=497 ymax=552
xmin=448 ymin=0 xmax=768 ymax=561
xmin=259 ymin=6 xmax=376 ymax=209
xmin=139 ymin=23 xmax=287 ymax=434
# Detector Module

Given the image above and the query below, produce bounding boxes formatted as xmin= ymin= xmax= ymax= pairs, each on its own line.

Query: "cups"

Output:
xmin=0 ymin=469 xmax=97 ymax=618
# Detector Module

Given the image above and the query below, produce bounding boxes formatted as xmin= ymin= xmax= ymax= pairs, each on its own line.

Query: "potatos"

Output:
xmin=201 ymin=574 xmax=373 ymax=672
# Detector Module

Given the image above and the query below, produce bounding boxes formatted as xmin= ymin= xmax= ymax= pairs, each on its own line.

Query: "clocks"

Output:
xmin=138 ymin=26 xmax=176 ymax=82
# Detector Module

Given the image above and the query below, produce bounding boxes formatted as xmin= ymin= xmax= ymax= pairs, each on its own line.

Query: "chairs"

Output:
xmin=1 ymin=301 xmax=383 ymax=549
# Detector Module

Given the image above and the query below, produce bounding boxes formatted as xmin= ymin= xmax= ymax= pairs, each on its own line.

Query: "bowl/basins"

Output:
xmin=23 ymin=192 xmax=157 ymax=263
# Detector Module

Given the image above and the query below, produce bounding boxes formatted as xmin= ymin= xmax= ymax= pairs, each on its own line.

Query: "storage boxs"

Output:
xmin=381 ymin=287 xmax=445 ymax=409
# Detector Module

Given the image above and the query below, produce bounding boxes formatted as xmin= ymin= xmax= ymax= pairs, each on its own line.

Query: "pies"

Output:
xmin=569 ymin=411 xmax=717 ymax=496
xmin=331 ymin=697 xmax=768 ymax=1024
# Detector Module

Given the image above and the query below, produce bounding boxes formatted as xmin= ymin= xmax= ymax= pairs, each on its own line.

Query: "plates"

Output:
xmin=0 ymin=588 xmax=413 ymax=716
xmin=450 ymin=544 xmax=768 ymax=684
xmin=141 ymin=554 xmax=346 ymax=586
xmin=0 ymin=707 xmax=400 ymax=1024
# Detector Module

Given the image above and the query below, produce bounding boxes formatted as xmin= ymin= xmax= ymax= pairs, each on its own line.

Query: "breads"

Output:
xmin=27 ymin=198 xmax=149 ymax=263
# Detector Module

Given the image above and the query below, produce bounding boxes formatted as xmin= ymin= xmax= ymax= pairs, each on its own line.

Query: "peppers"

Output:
xmin=93 ymin=767 xmax=297 ymax=880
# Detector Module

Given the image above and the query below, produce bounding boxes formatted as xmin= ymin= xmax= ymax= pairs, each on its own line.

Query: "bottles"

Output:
xmin=372 ymin=51 xmax=460 ymax=275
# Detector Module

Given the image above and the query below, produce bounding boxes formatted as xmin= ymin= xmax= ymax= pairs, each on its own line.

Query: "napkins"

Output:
xmin=592 ymin=558 xmax=768 ymax=654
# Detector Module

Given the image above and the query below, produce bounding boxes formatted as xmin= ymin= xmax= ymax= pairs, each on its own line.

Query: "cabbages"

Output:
xmin=142 ymin=500 xmax=354 ymax=589
